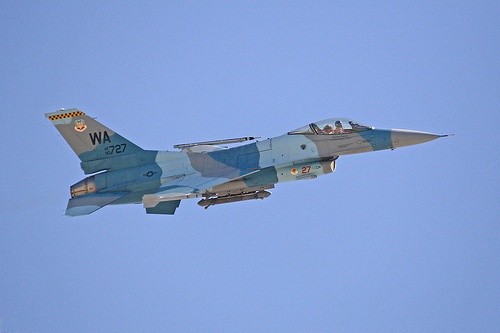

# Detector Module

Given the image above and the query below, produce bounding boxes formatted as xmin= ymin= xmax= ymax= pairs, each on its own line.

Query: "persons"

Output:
xmin=335 ymin=120 xmax=343 ymax=130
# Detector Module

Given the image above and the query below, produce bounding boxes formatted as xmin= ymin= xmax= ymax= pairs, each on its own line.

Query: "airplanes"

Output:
xmin=46 ymin=107 xmax=450 ymax=215
